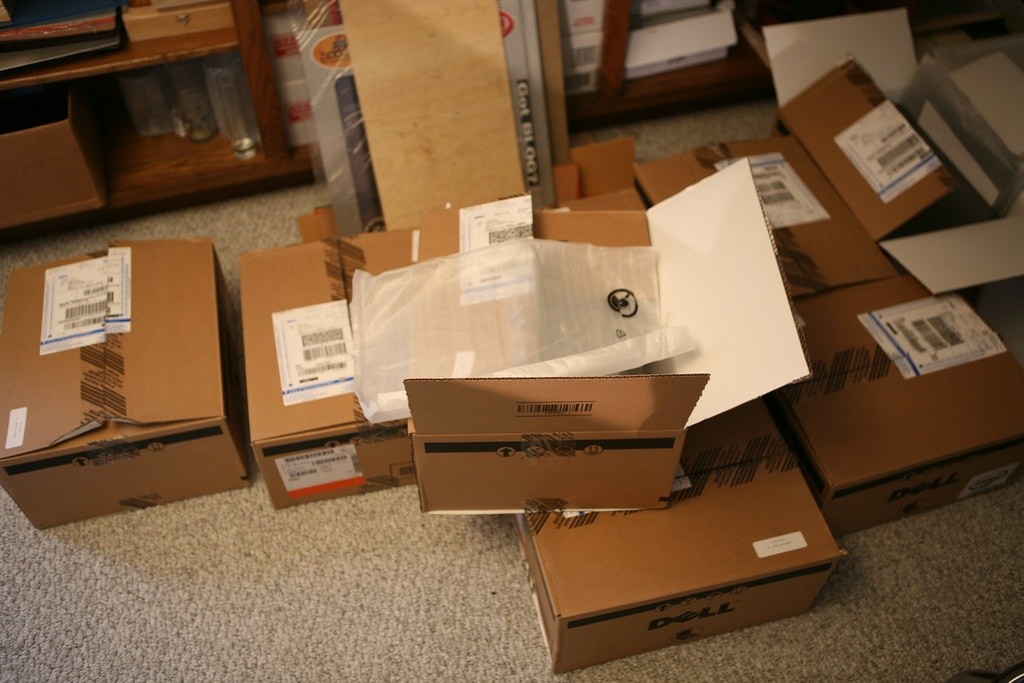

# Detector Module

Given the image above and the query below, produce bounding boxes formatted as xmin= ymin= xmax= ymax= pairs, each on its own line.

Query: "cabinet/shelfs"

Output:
xmin=0 ymin=0 xmax=771 ymax=244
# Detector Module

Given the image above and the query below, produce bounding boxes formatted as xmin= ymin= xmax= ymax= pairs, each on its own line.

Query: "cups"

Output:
xmin=118 ymin=54 xmax=260 ymax=158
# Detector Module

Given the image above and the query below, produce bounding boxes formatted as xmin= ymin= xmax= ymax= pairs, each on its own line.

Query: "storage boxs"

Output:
xmin=0 ymin=82 xmax=109 ymax=228
xmin=634 ymin=134 xmax=899 ymax=300
xmin=406 ymin=155 xmax=813 ymax=516
xmin=239 ymin=226 xmax=421 ymax=511
xmin=762 ymin=9 xmax=1024 ymax=295
xmin=512 ymin=399 xmax=848 ymax=675
xmin=770 ymin=273 xmax=1024 ymax=539
xmin=0 ymin=238 xmax=253 ymax=530
xmin=553 ymin=137 xmax=646 ymax=211
